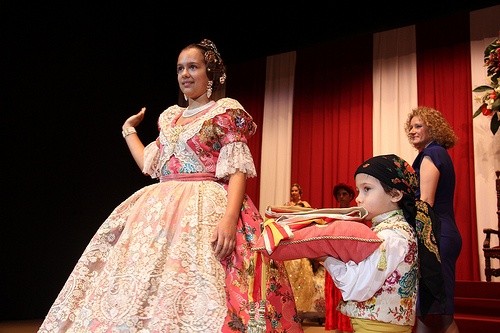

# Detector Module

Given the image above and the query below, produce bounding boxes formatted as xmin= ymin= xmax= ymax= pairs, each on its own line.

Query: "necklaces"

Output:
xmin=183 ymin=100 xmax=215 ymax=118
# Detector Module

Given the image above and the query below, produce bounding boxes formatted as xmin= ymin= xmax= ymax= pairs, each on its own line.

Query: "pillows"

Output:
xmin=250 ymin=219 xmax=383 ymax=262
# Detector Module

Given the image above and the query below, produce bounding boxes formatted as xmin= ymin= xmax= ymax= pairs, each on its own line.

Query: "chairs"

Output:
xmin=482 ymin=171 xmax=500 ymax=282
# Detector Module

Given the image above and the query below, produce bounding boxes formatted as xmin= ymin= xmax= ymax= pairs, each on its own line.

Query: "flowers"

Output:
xmin=472 ymin=38 xmax=500 ymax=136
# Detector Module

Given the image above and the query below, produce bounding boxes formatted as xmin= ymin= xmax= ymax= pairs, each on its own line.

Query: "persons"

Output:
xmin=405 ymin=106 xmax=462 ymax=333
xmin=312 ymin=154 xmax=419 ymax=333
xmin=35 ymin=39 xmax=304 ymax=333
xmin=316 ymin=183 xmax=355 ymax=317
xmin=274 ymin=184 xmax=315 ymax=312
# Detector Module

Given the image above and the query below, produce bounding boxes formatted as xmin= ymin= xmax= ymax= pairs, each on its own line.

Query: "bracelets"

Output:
xmin=122 ymin=127 xmax=137 ymax=137
